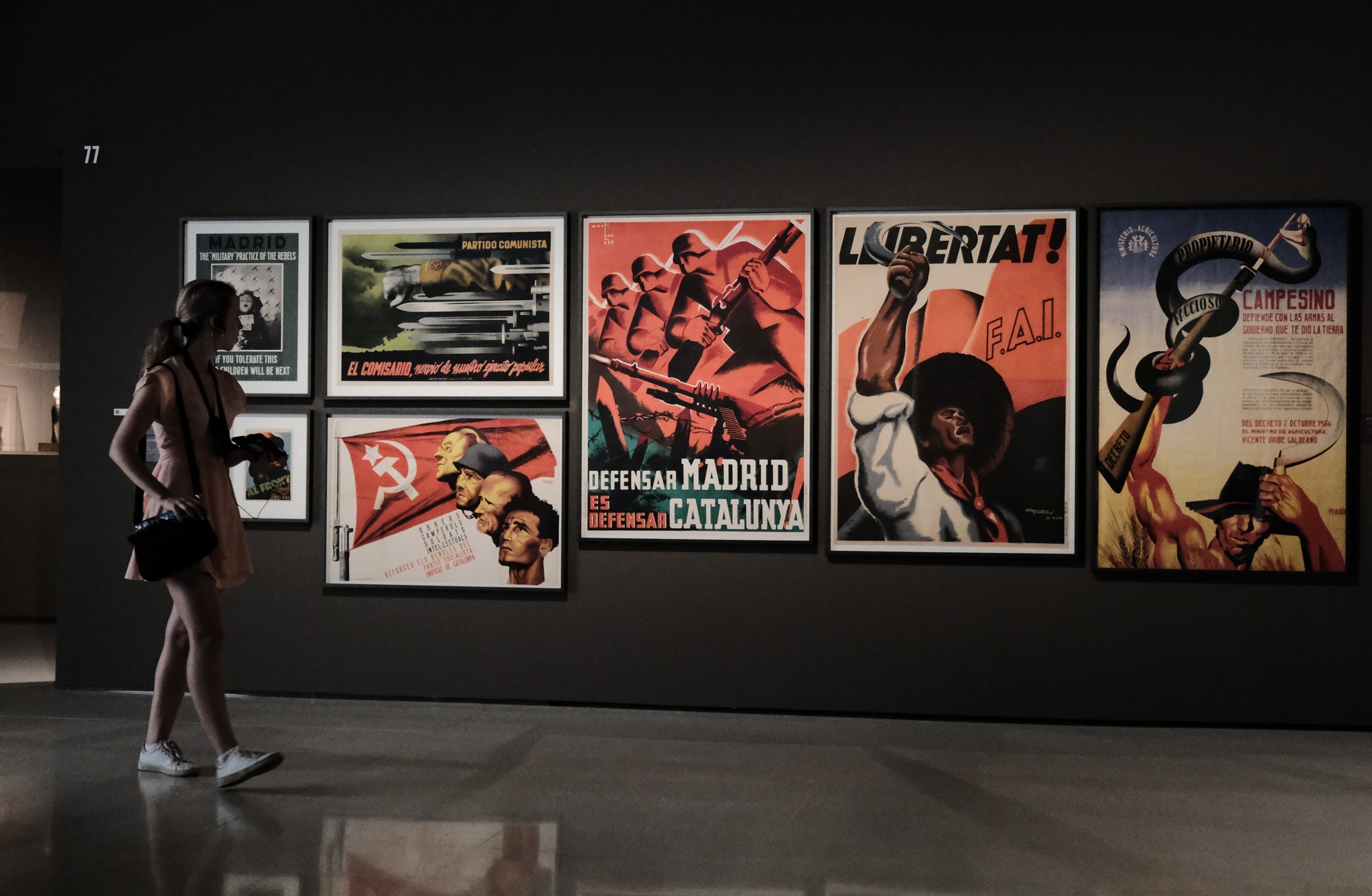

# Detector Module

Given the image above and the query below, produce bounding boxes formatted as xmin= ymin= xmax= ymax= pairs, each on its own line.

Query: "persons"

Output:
xmin=107 ymin=279 xmax=285 ymax=789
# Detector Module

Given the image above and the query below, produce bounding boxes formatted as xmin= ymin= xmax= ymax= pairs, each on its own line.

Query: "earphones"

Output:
xmin=213 ymin=318 xmax=225 ymax=331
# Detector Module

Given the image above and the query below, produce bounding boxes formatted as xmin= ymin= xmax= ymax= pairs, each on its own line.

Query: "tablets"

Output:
xmin=232 ymin=434 xmax=287 ymax=461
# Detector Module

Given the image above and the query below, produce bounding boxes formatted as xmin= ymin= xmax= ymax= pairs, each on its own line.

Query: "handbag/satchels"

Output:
xmin=133 ymin=508 xmax=217 ymax=583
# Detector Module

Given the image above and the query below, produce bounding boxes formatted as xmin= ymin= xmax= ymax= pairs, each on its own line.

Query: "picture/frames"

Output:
xmin=570 ymin=205 xmax=821 ymax=559
xmin=819 ymin=197 xmax=1088 ymax=572
xmin=173 ymin=218 xmax=318 ymax=533
xmin=312 ymin=410 xmax=574 ymax=599
xmin=319 ymin=209 xmax=573 ymax=410
xmin=1093 ymin=200 xmax=1359 ymax=581
xmin=216 ymin=806 xmax=564 ymax=896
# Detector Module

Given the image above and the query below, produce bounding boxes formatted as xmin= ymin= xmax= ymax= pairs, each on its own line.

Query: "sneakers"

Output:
xmin=137 ymin=739 xmax=198 ymax=777
xmin=215 ymin=745 xmax=284 ymax=787
